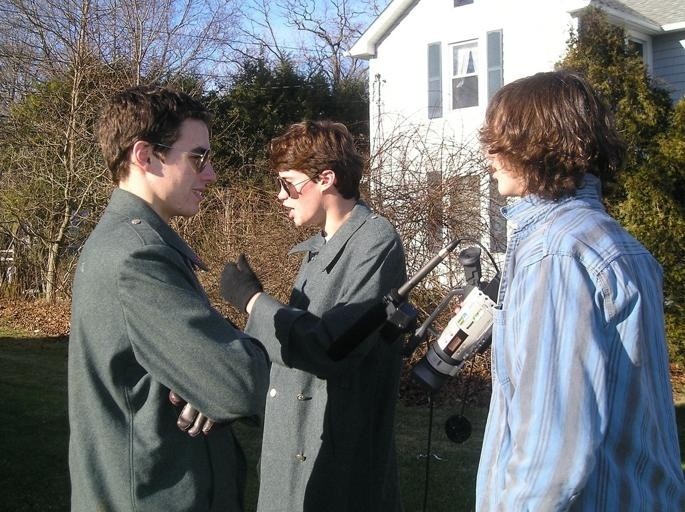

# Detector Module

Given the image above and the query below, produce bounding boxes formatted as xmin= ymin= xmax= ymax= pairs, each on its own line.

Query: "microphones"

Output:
xmin=328 ymin=241 xmax=465 ymax=363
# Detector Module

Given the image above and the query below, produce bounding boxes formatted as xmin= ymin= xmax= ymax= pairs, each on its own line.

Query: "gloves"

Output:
xmin=218 ymin=253 xmax=263 ymax=314
xmin=165 ymin=389 xmax=218 ymax=439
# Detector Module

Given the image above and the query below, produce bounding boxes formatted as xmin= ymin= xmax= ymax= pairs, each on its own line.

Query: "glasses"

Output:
xmin=276 ymin=172 xmax=320 ymax=198
xmin=152 ymin=141 xmax=214 ymax=174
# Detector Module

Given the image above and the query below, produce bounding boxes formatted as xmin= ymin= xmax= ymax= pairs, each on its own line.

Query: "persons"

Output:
xmin=473 ymin=70 xmax=685 ymax=512
xmin=66 ymin=82 xmax=272 ymax=510
xmin=220 ymin=116 xmax=412 ymax=511
